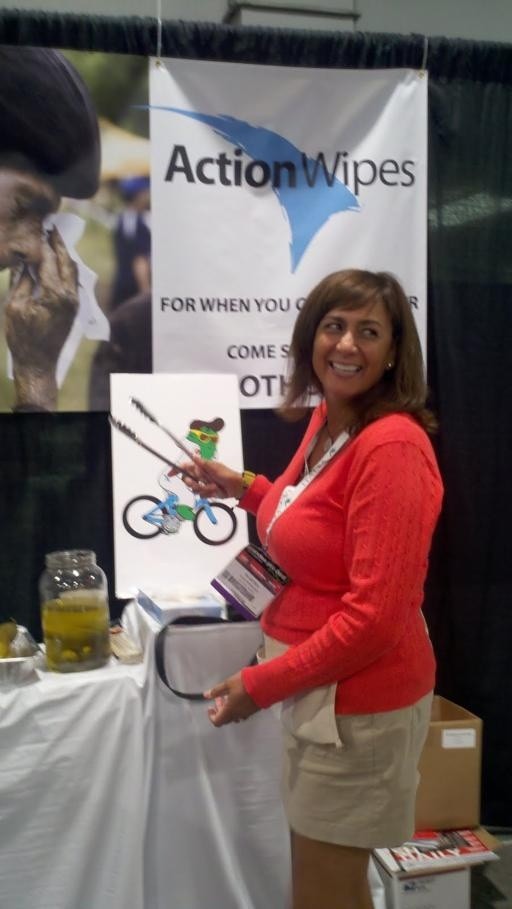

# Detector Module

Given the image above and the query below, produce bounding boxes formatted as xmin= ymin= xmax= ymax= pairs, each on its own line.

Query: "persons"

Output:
xmin=168 ymin=268 xmax=446 ymax=908
xmin=1 ymin=46 xmax=100 ymax=412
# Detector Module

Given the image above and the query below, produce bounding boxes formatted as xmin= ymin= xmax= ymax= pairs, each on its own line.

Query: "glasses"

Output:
xmin=189 ymin=428 xmax=219 ymax=444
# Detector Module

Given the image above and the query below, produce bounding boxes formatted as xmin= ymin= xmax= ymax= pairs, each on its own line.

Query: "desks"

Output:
xmin=0 ymin=655 xmax=292 ymax=908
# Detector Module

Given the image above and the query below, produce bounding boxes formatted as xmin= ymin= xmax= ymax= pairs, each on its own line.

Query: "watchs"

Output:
xmin=236 ymin=470 xmax=257 ymax=499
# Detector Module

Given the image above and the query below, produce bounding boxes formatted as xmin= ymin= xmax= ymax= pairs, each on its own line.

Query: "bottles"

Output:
xmin=41 ymin=549 xmax=112 ymax=674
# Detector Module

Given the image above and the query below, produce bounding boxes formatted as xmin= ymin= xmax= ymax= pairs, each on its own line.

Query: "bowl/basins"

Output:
xmin=0 ymin=657 xmax=35 ymax=686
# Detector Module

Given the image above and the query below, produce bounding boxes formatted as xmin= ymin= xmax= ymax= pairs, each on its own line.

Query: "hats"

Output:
xmin=1 ymin=43 xmax=101 ymax=199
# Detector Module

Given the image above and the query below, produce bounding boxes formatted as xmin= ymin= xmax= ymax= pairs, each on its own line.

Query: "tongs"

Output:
xmin=106 ymin=397 xmax=225 ymax=500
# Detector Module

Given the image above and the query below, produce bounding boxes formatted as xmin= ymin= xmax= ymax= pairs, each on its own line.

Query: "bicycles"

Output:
xmin=123 ymin=473 xmax=242 ymax=545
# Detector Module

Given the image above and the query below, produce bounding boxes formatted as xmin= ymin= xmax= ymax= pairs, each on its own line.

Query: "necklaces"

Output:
xmin=322 ymin=426 xmax=339 ymax=453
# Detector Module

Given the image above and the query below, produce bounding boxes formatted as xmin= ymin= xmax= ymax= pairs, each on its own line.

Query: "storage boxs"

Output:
xmin=132 ymin=600 xmax=264 ymax=701
xmin=409 ymin=694 xmax=483 ymax=831
xmin=367 ymin=854 xmax=471 ymax=909
